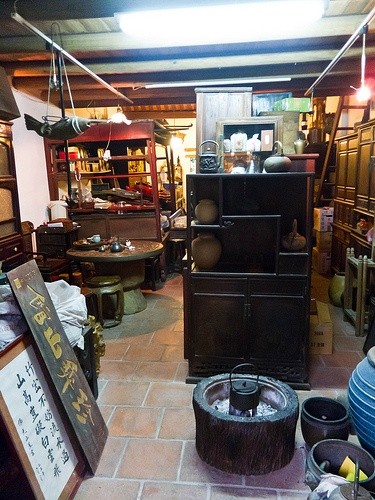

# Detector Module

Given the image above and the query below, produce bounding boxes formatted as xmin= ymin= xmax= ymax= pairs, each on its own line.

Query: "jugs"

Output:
xmin=293 ymin=137 xmax=309 ymax=154
xmin=110 ymin=242 xmax=123 ymax=252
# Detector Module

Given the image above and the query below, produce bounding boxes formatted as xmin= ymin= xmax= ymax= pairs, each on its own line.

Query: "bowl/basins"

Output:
xmin=301 ymin=397 xmax=350 ymax=448
xmin=305 ymin=439 xmax=375 ymax=491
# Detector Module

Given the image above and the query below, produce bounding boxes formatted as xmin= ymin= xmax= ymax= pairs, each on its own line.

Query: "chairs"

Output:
xmin=22 ymin=230 xmax=68 ymax=282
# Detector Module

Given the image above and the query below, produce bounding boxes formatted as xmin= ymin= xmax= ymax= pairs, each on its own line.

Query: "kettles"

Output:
xmin=229 ymin=363 xmax=264 ymax=411
xmin=198 ymin=140 xmax=222 ymax=173
xmin=263 ymin=141 xmax=292 ymax=173
xmin=329 ymin=458 xmax=374 ymax=500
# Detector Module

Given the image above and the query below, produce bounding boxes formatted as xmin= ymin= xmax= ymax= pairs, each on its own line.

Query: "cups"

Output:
xmin=91 ymin=235 xmax=100 ymax=242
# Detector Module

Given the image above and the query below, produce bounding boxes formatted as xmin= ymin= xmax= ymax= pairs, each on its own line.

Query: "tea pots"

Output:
xmin=282 ymin=219 xmax=306 ymax=251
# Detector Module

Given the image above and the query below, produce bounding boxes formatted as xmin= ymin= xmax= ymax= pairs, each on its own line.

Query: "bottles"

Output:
xmin=192 ymin=233 xmax=221 ymax=270
xmin=329 ymin=272 xmax=354 ymax=307
xmin=195 ymin=199 xmax=218 ymax=224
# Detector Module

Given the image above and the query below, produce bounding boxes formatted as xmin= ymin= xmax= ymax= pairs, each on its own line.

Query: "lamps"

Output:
xmin=113 ymin=0 xmax=328 ymax=41
xmin=108 ymin=95 xmax=132 ymax=125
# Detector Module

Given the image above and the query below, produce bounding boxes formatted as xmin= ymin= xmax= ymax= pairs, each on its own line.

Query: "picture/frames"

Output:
xmin=0 ymin=330 xmax=87 ymax=500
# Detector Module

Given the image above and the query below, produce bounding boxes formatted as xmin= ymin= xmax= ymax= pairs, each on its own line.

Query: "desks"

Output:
xmin=66 ymin=239 xmax=164 ymax=315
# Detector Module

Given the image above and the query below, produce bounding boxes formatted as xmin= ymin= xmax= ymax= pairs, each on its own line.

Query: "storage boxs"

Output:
xmin=313 ymin=247 xmax=332 ymax=276
xmin=309 ymin=301 xmax=333 ymax=355
xmin=313 ymin=207 xmax=334 ymax=231
xmin=316 ymin=231 xmax=332 ymax=252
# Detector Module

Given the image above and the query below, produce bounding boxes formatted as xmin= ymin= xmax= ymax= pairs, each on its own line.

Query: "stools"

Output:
xmin=86 ymin=275 xmax=125 ymax=327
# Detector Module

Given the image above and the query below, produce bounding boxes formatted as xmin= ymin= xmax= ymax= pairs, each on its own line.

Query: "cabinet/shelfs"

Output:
xmin=184 ymin=173 xmax=314 ymax=390
xmin=42 ymin=121 xmax=160 ymax=242
xmin=329 ymin=119 xmax=375 ymax=282
